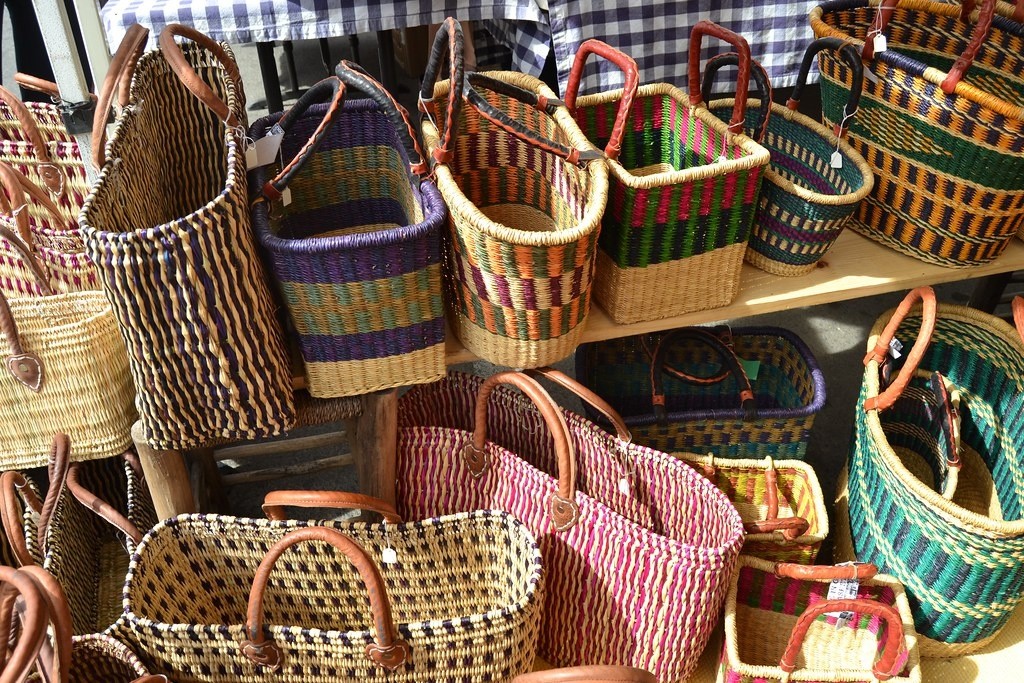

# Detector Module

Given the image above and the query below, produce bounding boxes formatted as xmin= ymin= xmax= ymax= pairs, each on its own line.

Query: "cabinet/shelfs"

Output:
xmin=127 ymin=210 xmax=1024 ymax=681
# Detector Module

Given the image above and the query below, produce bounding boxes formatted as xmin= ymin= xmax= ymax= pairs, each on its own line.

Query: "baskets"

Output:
xmin=0 ymin=71 xmax=166 ymax=683
xmin=122 ymin=489 xmax=544 ymax=683
xmin=668 ymin=449 xmax=829 ymax=565
xmin=562 ymin=20 xmax=771 ymax=326
xmin=809 ymin=0 xmax=1023 ymax=270
xmin=246 ymin=60 xmax=448 ymax=398
xmin=882 ymin=361 xmax=961 ymax=502
xmin=388 ymin=366 xmax=748 ymax=680
xmin=850 ymin=285 xmax=1024 ymax=655
xmin=702 ymin=36 xmax=874 ymax=276
xmin=77 ymin=22 xmax=296 ymax=451
xmin=572 ymin=325 xmax=825 ymax=460
xmin=712 ymin=554 xmax=925 ymax=682
xmin=417 ymin=17 xmax=610 ymax=371
